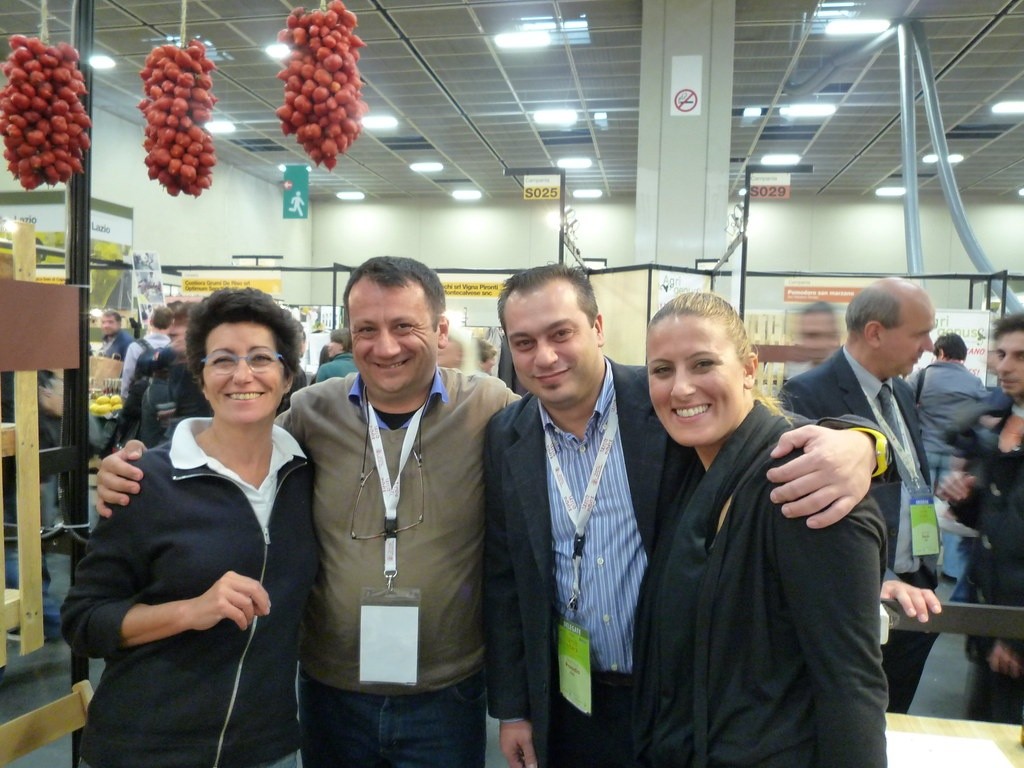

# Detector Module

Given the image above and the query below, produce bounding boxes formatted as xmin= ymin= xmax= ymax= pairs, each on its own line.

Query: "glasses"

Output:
xmin=200 ymin=349 xmax=294 ymax=375
xmin=350 ymin=448 xmax=425 ymax=540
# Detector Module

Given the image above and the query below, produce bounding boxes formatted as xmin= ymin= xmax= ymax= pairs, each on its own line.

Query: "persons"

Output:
xmin=118 ymin=306 xmax=175 ymax=415
xmin=934 ymin=307 xmax=1024 ymax=724
xmin=57 ymin=285 xmax=321 ymax=767
xmin=472 ymin=337 xmax=501 ymax=378
xmin=268 ymin=318 xmax=309 ymax=417
xmin=901 ymin=329 xmax=996 ymax=592
xmin=783 ymin=291 xmax=842 ymax=385
xmin=479 ymin=260 xmax=907 ymax=768
xmin=98 ymin=306 xmax=135 ymax=364
xmin=1 ymin=364 xmax=86 ymax=649
xmin=93 ymin=254 xmax=528 ymax=768
xmin=136 ymin=300 xmax=215 ymax=454
xmin=308 ymin=346 xmax=341 ymax=389
xmin=160 ymin=297 xmax=186 ymax=357
xmin=314 ymin=325 xmax=364 ymax=384
xmin=623 ymin=289 xmax=895 ymax=768
xmin=774 ymin=274 xmax=950 ymax=715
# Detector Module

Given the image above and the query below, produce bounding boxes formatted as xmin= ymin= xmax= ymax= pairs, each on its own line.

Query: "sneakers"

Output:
xmin=6 ymin=628 xmax=48 ymax=642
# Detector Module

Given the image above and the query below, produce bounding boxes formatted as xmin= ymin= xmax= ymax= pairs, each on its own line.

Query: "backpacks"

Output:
xmin=123 ymin=337 xmax=164 ymax=421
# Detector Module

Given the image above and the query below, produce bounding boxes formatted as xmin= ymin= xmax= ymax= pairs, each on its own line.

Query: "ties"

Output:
xmin=880 ymin=385 xmax=919 ymax=497
